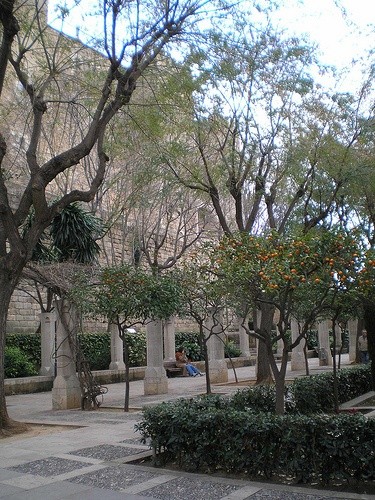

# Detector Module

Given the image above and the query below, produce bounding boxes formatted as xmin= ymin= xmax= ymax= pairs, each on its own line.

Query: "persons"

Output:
xmin=175 ymin=346 xmax=191 ymax=377
xmin=358 ymin=327 xmax=370 ymax=365
xmin=184 ymin=349 xmax=206 ymax=376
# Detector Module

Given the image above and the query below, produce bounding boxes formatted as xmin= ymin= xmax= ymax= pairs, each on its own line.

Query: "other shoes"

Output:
xmin=193 ymin=373 xmax=198 ymax=377
xmin=200 ymin=373 xmax=205 ymax=376
